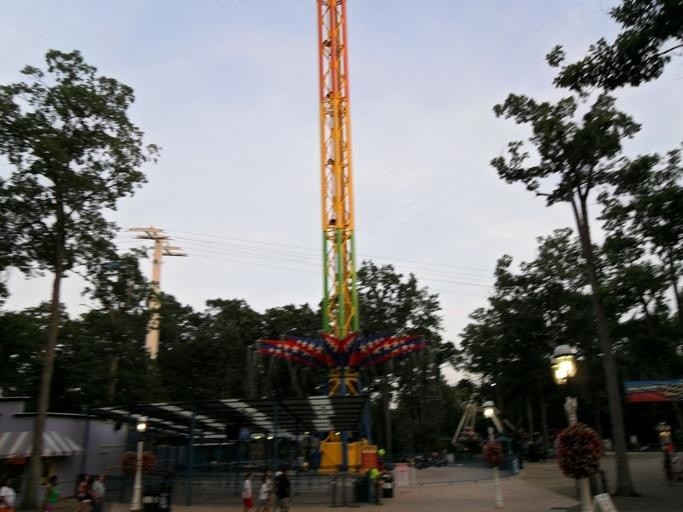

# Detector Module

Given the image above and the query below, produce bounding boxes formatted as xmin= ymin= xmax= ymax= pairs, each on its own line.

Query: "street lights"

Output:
xmin=480 ymin=399 xmax=505 ymax=507
xmin=657 ymin=422 xmax=675 ymax=477
xmin=128 ymin=416 xmax=148 ymax=510
xmin=548 ymin=341 xmax=593 ymax=512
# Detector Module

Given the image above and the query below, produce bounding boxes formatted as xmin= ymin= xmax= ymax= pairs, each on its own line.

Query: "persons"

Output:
xmin=660 ymin=439 xmax=674 ymax=483
xmin=0 ymin=472 xmax=106 ymax=512
xmin=368 ymin=468 xmax=385 ymax=503
xmin=242 ymin=465 xmax=292 ymax=512
